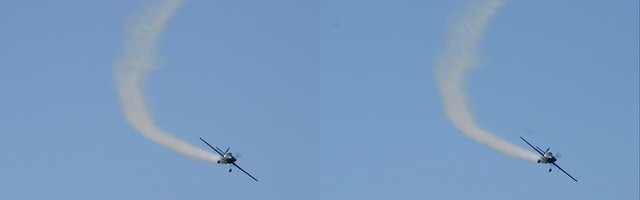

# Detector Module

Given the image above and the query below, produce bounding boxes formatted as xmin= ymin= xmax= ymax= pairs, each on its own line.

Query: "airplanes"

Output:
xmin=200 ymin=138 xmax=258 ymax=181
xmin=520 ymin=137 xmax=577 ymax=182
